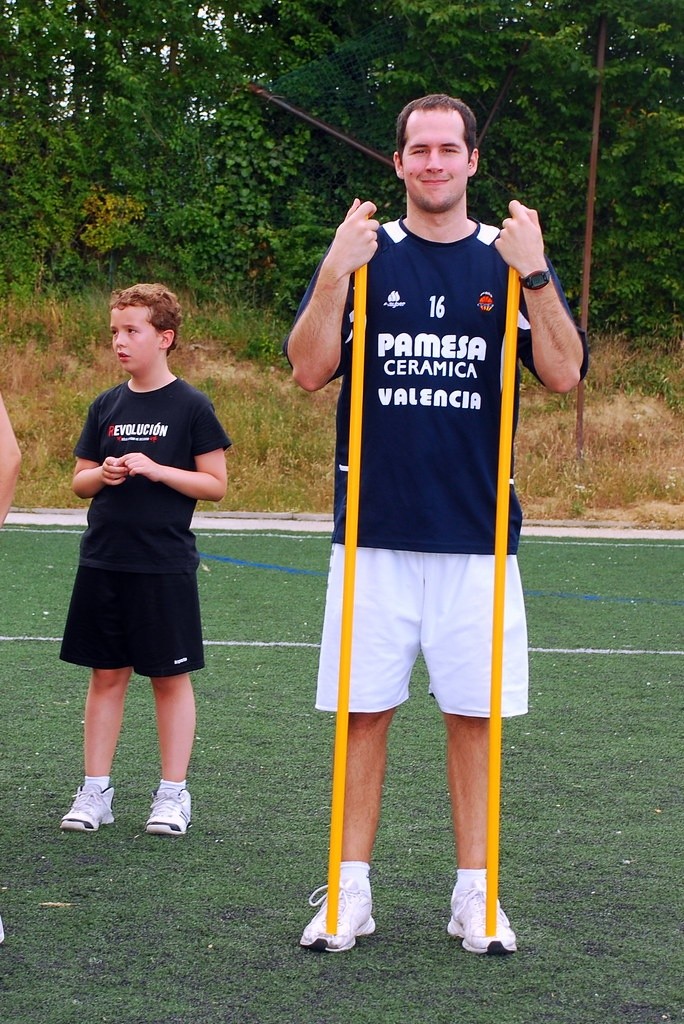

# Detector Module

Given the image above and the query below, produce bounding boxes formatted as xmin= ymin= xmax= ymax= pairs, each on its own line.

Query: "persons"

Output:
xmin=58 ymin=281 xmax=233 ymax=837
xmin=1 ymin=391 xmax=22 ymax=531
xmin=282 ymin=91 xmax=591 ymax=960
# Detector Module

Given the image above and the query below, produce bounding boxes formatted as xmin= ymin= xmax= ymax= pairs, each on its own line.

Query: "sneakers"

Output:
xmin=297 ymin=887 xmax=517 ymax=956
xmin=60 ymin=784 xmax=193 ymax=836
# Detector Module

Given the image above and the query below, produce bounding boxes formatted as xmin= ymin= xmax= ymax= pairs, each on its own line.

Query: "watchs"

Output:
xmin=518 ymin=266 xmax=552 ymax=290
